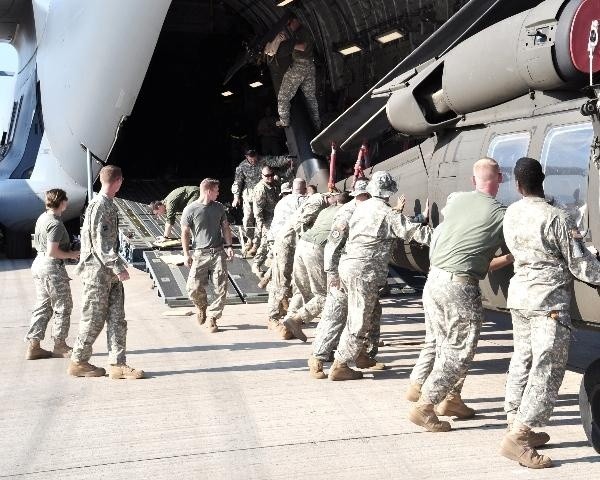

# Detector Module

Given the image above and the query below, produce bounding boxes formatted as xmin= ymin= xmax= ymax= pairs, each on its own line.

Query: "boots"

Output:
xmin=407 ymin=382 xmax=421 ymax=401
xmin=357 ymin=355 xmax=385 ymax=370
xmin=245 ymin=241 xmax=252 ymax=251
xmin=198 ymin=306 xmax=207 ymax=324
xmin=499 ymin=420 xmax=552 ymax=469
xmin=508 ymin=417 xmax=550 ymax=449
xmin=109 ymin=365 xmax=145 ymax=379
xmin=308 ymin=357 xmax=325 ymax=379
xmin=268 ymin=318 xmax=283 ymax=332
xmin=282 ymin=314 xmax=307 ymax=342
xmin=26 ymin=339 xmax=51 ymax=360
xmin=67 ymin=362 xmax=106 ymax=377
xmin=276 ymin=118 xmax=291 ymax=127
xmin=249 ymin=244 xmax=259 ymax=255
xmin=208 ymin=317 xmax=218 ymax=333
xmin=438 ymin=394 xmax=475 ymax=419
xmin=51 ymin=339 xmax=73 ymax=357
xmin=409 ymin=401 xmax=452 ymax=432
xmin=328 ymin=359 xmax=363 ymax=380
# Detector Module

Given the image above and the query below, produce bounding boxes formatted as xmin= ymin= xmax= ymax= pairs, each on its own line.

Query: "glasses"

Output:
xmin=263 ymin=173 xmax=274 ymax=177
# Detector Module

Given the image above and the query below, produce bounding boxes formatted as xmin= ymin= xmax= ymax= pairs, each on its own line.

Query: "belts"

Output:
xmin=202 ymin=247 xmax=224 ymax=253
xmin=429 ymin=269 xmax=468 ymax=282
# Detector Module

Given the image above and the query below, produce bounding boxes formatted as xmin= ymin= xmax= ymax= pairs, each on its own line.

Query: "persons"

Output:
xmin=308 ymin=177 xmax=371 ymax=379
xmin=267 ymin=193 xmax=337 ymax=333
xmin=67 ymin=164 xmax=147 ymax=380
xmin=149 ymin=185 xmax=201 ymax=244
xmin=280 ymin=191 xmax=354 ymax=343
xmin=257 ymin=177 xmax=317 ymax=296
xmin=328 ymin=169 xmax=436 ymax=382
xmin=247 ymin=162 xmax=296 ymax=259
xmin=405 ymin=156 xmax=515 ymax=433
xmin=354 ymin=176 xmax=387 ymax=370
xmin=264 ymin=14 xmax=323 ymax=129
xmin=24 ymin=188 xmax=81 ymax=361
xmin=179 ymin=177 xmax=234 ymax=333
xmin=231 ymin=149 xmax=293 ymax=252
xmin=497 ymin=156 xmax=600 ymax=469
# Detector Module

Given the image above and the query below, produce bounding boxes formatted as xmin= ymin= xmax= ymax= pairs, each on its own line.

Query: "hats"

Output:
xmin=279 ymin=182 xmax=292 ymax=196
xmin=349 ymin=180 xmax=370 ymax=196
xmin=365 ymin=171 xmax=398 ymax=197
